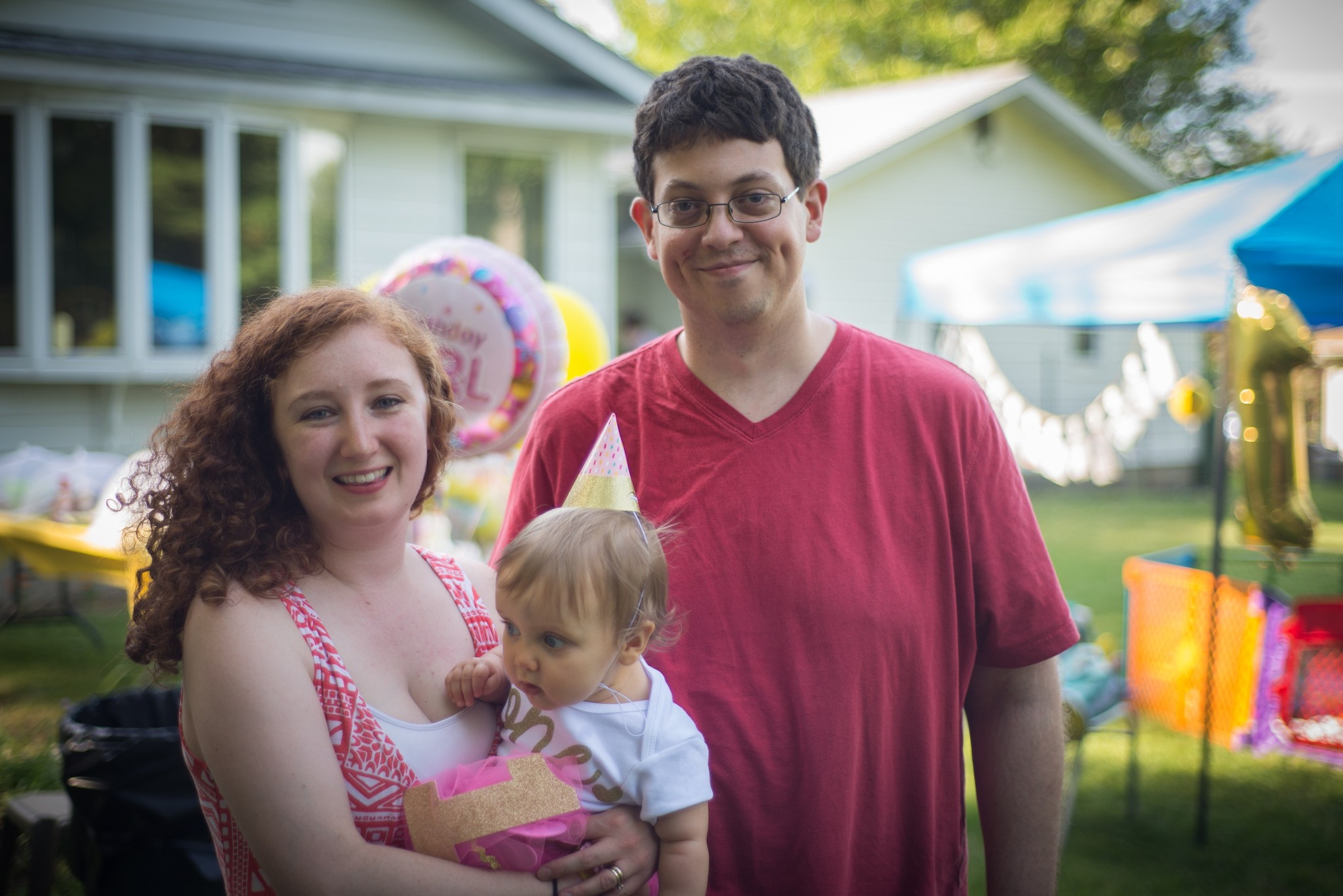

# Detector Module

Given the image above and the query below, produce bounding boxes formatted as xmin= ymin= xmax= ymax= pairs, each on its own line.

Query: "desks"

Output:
xmin=0 ymin=509 xmax=156 ymax=621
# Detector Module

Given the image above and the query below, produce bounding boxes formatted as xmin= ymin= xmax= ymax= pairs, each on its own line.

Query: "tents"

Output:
xmin=904 ymin=157 xmax=1343 ymax=842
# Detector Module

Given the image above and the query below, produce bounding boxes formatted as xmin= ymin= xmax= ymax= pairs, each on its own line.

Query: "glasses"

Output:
xmin=648 ymin=184 xmax=802 ymax=229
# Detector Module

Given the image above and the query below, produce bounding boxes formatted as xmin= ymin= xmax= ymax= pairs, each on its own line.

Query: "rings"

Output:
xmin=607 ymin=864 xmax=624 ymax=891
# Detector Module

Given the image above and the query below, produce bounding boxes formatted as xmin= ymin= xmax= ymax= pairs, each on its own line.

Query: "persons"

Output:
xmin=484 ymin=50 xmax=1083 ymax=896
xmin=103 ymin=285 xmax=660 ymax=896
xmin=447 ymin=505 xmax=715 ymax=896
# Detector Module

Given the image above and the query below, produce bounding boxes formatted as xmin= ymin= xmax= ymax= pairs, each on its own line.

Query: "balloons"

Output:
xmin=365 ymin=236 xmax=565 ymax=465
xmin=546 ymin=281 xmax=612 ymax=380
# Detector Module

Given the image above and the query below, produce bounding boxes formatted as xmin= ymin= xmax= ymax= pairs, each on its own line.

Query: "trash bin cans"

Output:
xmin=57 ymin=685 xmax=226 ymax=895
